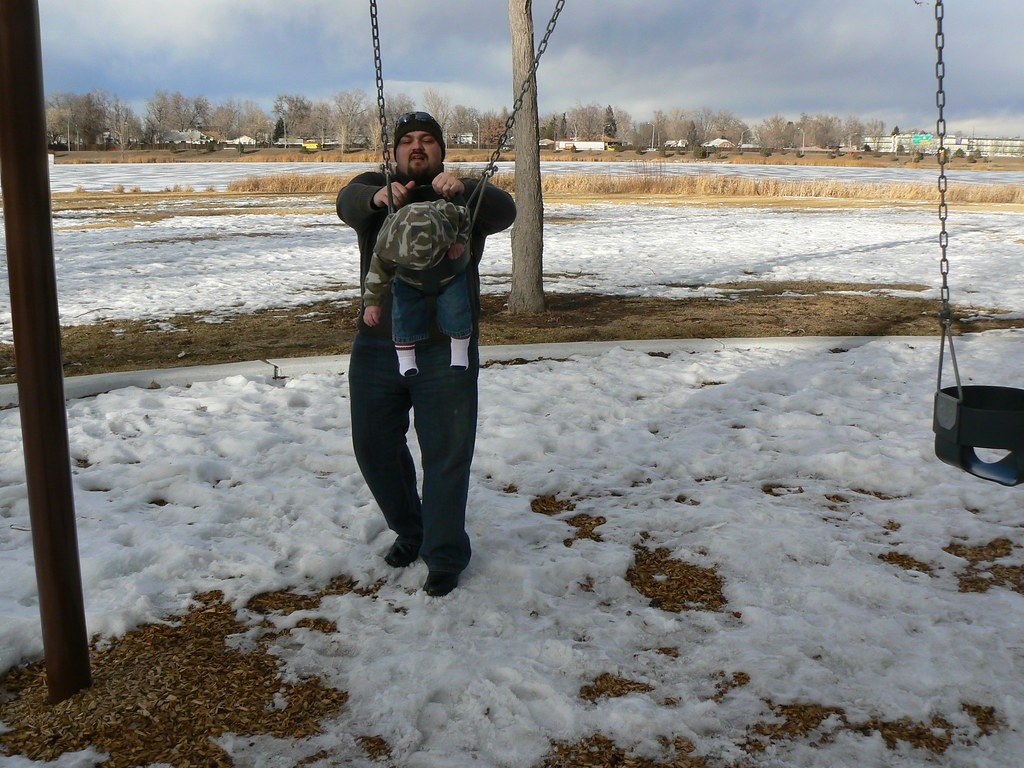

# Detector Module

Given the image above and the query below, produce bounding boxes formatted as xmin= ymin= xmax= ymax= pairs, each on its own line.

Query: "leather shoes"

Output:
xmin=385 ymin=536 xmax=422 ymax=567
xmin=423 ymin=571 xmax=458 ymax=596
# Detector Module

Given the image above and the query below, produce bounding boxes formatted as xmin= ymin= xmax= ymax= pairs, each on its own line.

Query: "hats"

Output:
xmin=394 ymin=112 xmax=446 ymax=162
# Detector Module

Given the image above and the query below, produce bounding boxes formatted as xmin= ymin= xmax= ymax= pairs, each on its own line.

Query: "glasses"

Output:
xmin=396 ymin=112 xmax=441 ymax=130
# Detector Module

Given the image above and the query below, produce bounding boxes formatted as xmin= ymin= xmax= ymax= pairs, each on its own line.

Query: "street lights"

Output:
xmin=797 ymin=127 xmax=808 ymax=157
xmin=603 ymin=125 xmax=610 ymax=141
xmin=647 ymin=123 xmax=659 ymax=150
xmin=741 ymin=128 xmax=752 ymax=148
xmin=472 ymin=119 xmax=481 ymax=150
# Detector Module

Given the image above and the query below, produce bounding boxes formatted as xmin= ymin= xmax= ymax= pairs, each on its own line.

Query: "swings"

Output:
xmin=929 ymin=0 xmax=1024 ymax=488
xmin=367 ymin=1 xmax=565 ymax=300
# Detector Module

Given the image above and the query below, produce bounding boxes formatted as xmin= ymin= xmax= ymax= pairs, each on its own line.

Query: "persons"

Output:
xmin=336 ymin=110 xmax=517 ymax=597
